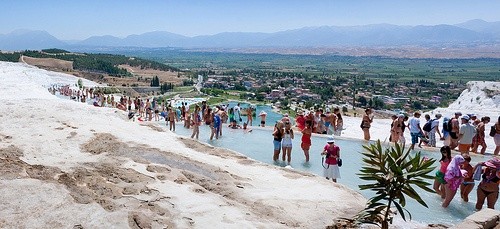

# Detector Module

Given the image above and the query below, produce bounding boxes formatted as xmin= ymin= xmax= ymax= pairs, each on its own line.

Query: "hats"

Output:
xmin=283 ymin=107 xmax=331 ymax=117
xmin=392 ymin=111 xmax=476 ymax=121
xmin=484 ymin=157 xmax=500 ymax=169
xmin=326 ymin=136 xmax=335 ymax=142
xmin=212 ymin=107 xmax=219 ymax=113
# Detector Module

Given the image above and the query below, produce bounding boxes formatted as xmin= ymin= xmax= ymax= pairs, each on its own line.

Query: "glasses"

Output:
xmin=442 ymin=151 xmax=447 ymax=153
xmin=328 ymin=142 xmax=333 ymax=144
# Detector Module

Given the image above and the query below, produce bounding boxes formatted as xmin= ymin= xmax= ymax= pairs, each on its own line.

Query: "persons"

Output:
xmin=448 ymin=111 xmax=462 ymax=150
xmin=301 ymin=119 xmax=312 ymax=162
xmin=471 ymin=157 xmax=500 ymax=210
xmin=272 ymin=121 xmax=284 ymax=162
xmin=472 ymin=116 xmax=491 ymax=154
xmin=433 ymin=146 xmax=452 ymax=198
xmin=274 ymin=105 xmax=343 ymax=138
xmin=361 ymin=108 xmax=374 ymax=141
xmin=441 ymin=155 xmax=468 ymax=207
xmin=321 ymin=136 xmax=340 ymax=183
xmin=281 ymin=121 xmax=294 ymax=164
xmin=493 ymin=116 xmax=500 ymax=155
xmin=458 ymin=114 xmax=477 ymax=157
xmin=387 ymin=112 xmax=485 ymax=149
xmin=459 ymin=155 xmax=475 ymax=203
xmin=47 ymin=82 xmax=267 ymax=142
